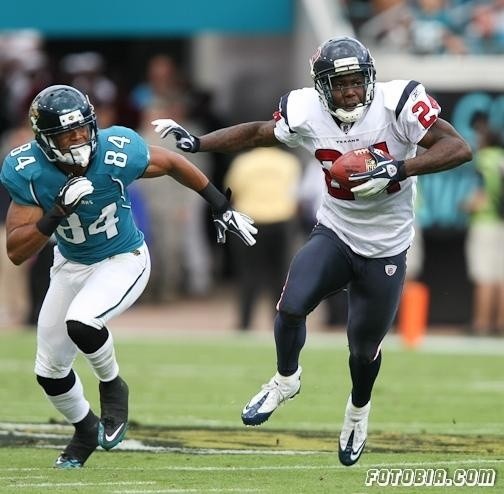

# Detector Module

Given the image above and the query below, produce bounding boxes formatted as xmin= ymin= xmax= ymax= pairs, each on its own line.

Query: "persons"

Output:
xmin=0 ymin=2 xmax=502 ymax=335
xmin=151 ymin=37 xmax=473 ymax=465
xmin=0 ymin=85 xmax=259 ymax=469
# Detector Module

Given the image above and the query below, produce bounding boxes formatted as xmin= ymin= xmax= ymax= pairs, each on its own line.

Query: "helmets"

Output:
xmin=307 ymin=35 xmax=378 ymax=125
xmin=27 ymin=83 xmax=100 ymax=171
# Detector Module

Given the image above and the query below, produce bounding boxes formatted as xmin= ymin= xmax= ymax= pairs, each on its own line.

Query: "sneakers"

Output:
xmin=52 ymin=415 xmax=101 ymax=472
xmin=336 ymin=392 xmax=373 ymax=467
xmin=240 ymin=363 xmax=305 ymax=427
xmin=90 ymin=377 xmax=132 ymax=451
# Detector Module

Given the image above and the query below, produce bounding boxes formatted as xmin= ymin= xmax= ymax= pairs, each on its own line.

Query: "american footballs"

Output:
xmin=330 ymin=148 xmax=397 ymax=192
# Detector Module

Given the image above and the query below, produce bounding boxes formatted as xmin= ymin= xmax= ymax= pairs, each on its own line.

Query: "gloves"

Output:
xmin=208 ymin=186 xmax=260 ymax=248
xmin=52 ymin=173 xmax=96 ymax=220
xmin=149 ymin=117 xmax=200 ymax=154
xmin=348 ymin=145 xmax=409 ymax=200
